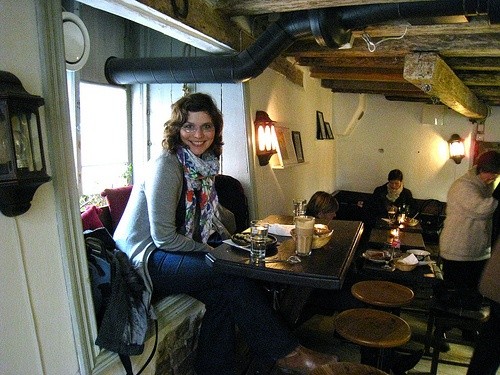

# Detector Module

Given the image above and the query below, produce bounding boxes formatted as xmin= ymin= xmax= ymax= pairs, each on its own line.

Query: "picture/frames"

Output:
xmin=324 ymin=122 xmax=334 ymax=139
xmin=315 ymin=111 xmax=327 ymax=140
xmin=292 ymin=131 xmax=305 ymax=162
xmin=275 ymin=126 xmax=298 ymax=164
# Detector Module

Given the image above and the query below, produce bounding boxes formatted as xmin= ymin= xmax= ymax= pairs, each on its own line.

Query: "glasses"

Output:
xmin=181 ymin=123 xmax=217 ymax=133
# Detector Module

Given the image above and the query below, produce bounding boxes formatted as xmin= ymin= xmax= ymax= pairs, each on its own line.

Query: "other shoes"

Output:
xmin=462 ymin=330 xmax=481 ymax=341
xmin=434 ymin=328 xmax=447 ymax=339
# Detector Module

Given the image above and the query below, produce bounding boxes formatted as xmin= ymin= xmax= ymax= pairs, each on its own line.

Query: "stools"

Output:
xmin=308 ymin=279 xmax=414 ymax=375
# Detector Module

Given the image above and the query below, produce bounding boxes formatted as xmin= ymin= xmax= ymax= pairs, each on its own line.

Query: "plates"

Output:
xmin=222 ymin=239 xmax=250 ymax=252
xmin=362 ymin=251 xmax=393 ymax=264
xmin=232 ymin=232 xmax=278 ymax=249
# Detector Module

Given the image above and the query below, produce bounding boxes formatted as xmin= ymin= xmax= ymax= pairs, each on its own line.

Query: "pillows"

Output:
xmin=101 ymin=187 xmax=132 ymax=226
xmin=81 ymin=205 xmax=111 ymax=234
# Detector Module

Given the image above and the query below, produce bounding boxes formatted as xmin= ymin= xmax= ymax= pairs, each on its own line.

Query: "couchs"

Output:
xmin=78 ymin=175 xmax=249 ymax=375
xmin=330 ymin=189 xmax=446 ymax=235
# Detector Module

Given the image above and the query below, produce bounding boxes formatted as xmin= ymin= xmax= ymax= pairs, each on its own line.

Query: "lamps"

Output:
xmin=254 ymin=109 xmax=278 ymax=166
xmin=0 ymin=67 xmax=53 ymax=217
xmin=447 ymin=134 xmax=465 ymax=164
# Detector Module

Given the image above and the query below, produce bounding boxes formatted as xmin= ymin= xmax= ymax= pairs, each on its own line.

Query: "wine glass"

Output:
xmin=387 ymin=206 xmax=396 ymax=228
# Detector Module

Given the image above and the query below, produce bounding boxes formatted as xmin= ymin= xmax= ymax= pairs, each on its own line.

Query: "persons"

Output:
xmin=306 ymin=191 xmax=339 ymax=220
xmin=435 ymin=151 xmax=500 ymax=342
xmin=113 ymin=93 xmax=339 ymax=375
xmin=372 ymin=170 xmax=413 ymax=229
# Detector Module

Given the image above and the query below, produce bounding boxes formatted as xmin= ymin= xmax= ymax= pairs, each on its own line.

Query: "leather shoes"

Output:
xmin=277 ymin=343 xmax=340 ymax=375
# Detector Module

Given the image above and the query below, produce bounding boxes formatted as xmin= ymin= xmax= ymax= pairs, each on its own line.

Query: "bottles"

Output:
xmin=397 ymin=203 xmax=406 ymax=224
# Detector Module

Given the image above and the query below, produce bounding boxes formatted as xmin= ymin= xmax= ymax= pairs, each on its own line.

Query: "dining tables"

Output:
xmin=369 ymin=216 xmax=425 ymax=250
xmin=204 ymin=213 xmax=364 ymax=375
xmin=356 ymin=248 xmax=442 ymax=375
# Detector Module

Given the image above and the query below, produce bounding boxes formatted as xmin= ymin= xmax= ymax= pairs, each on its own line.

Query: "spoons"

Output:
xmin=264 ymin=256 xmax=302 ymax=265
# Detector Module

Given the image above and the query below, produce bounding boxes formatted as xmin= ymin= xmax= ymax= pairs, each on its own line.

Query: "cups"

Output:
xmin=293 ymin=216 xmax=315 ymax=256
xmin=250 ymin=219 xmax=269 ymax=258
xmin=292 ymin=198 xmax=307 ymax=219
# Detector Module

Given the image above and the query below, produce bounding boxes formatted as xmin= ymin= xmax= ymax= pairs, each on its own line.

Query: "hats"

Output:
xmin=476 ymin=150 xmax=500 ymax=175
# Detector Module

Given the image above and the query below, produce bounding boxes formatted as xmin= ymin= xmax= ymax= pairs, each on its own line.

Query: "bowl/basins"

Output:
xmin=408 ymin=219 xmax=419 ymax=227
xmin=290 ymin=228 xmax=334 ymax=249
xmin=406 ymin=249 xmax=431 ymax=260
xmin=391 ymin=258 xmax=419 ymax=271
